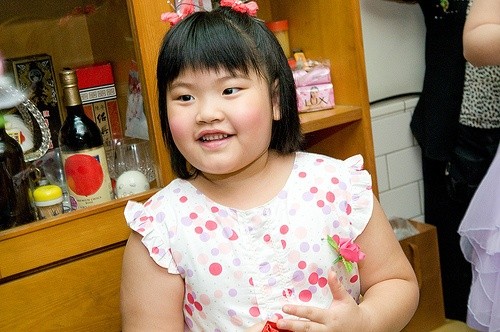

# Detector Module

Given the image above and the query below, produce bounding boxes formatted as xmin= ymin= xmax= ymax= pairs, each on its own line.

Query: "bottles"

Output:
xmin=34 ymin=187 xmax=64 ymax=222
xmin=57 ymin=67 xmax=115 ymax=212
xmin=264 ymin=20 xmax=291 ymax=60
xmin=0 ymin=114 xmax=38 ymax=234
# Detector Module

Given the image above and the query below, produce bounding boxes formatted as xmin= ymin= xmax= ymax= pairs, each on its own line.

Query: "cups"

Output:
xmin=33 ymin=161 xmax=49 ymax=186
xmin=115 ymin=139 xmax=156 ymax=186
xmin=43 ymin=147 xmax=68 ymax=202
xmin=102 ymin=134 xmax=134 ymax=181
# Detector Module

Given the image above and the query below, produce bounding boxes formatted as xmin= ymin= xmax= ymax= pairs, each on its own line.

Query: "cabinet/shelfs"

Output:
xmin=0 ymin=1 xmax=379 ymax=330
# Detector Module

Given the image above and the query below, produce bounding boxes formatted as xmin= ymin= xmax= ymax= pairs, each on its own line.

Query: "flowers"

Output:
xmin=325 ymin=233 xmax=367 ymax=273
xmin=160 ymin=0 xmax=260 ymax=28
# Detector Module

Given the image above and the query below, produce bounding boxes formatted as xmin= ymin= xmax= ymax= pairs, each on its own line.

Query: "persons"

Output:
xmin=119 ymin=0 xmax=420 ymax=332
xmin=409 ymin=0 xmax=500 ymax=332
xmin=306 ymin=87 xmax=323 ymax=106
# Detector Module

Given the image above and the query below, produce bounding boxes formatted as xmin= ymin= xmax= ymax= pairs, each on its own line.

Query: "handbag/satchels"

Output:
xmin=389 ymin=218 xmax=446 ymax=332
